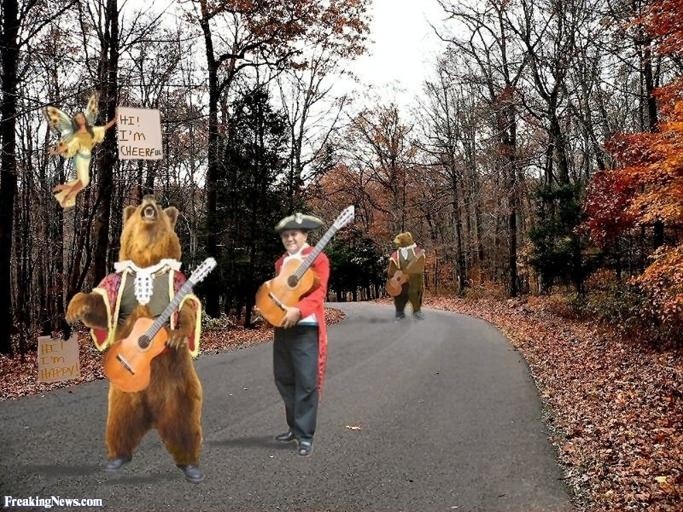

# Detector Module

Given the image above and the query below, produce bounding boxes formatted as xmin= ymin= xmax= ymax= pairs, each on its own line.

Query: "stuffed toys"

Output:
xmin=388 ymin=231 xmax=425 ymax=319
xmin=62 ymin=194 xmax=204 ymax=483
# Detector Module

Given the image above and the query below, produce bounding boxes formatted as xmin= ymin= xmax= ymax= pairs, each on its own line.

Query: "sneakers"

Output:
xmin=274 ymin=431 xmax=313 ymax=456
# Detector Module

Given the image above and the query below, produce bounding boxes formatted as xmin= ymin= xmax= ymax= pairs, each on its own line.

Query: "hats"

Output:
xmin=274 ymin=213 xmax=323 ymax=232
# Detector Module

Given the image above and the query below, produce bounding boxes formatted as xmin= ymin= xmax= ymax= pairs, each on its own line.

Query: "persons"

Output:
xmin=254 ymin=213 xmax=330 ymax=454
xmin=52 ymin=111 xmax=115 ymax=207
